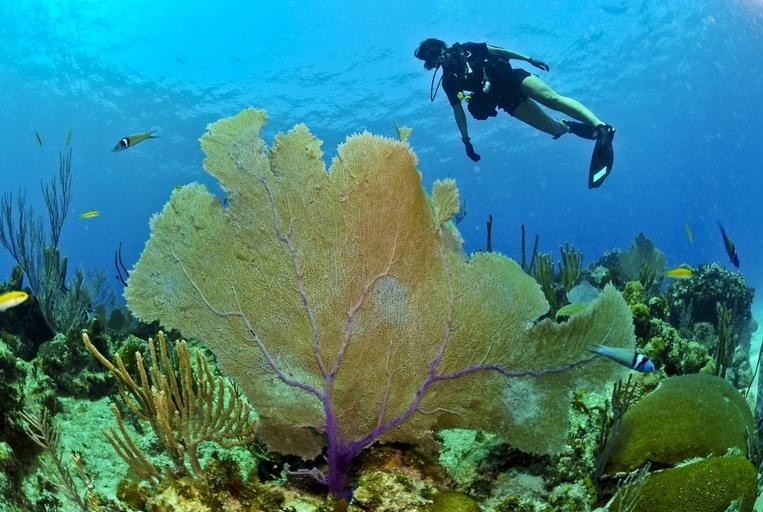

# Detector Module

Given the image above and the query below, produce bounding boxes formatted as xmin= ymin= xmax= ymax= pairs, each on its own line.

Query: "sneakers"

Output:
xmin=591 ymin=123 xmax=616 ymax=155
xmin=558 ymin=120 xmax=584 ymax=137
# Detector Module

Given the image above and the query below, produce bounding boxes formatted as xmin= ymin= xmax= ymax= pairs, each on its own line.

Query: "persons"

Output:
xmin=414 ymin=37 xmax=616 ymax=163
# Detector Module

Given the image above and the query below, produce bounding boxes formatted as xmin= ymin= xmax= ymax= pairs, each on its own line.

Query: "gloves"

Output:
xmin=528 ymin=55 xmax=551 ymax=73
xmin=461 ymin=135 xmax=481 ymax=163
